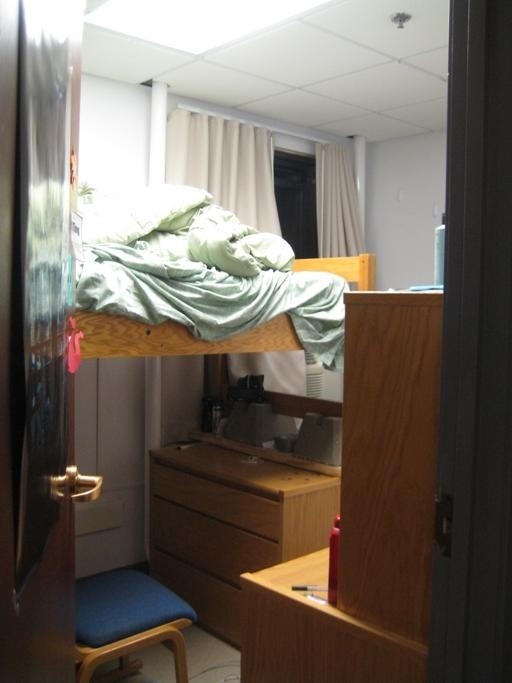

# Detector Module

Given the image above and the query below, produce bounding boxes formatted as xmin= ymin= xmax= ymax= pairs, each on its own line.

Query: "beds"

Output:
xmin=76 ymin=251 xmax=377 ymax=359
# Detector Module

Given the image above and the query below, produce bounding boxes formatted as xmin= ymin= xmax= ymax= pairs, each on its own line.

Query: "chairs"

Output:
xmin=76 ymin=566 xmax=197 ymax=682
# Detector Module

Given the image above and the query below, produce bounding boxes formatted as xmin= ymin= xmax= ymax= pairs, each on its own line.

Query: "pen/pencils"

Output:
xmin=177 ymin=443 xmax=202 ymax=450
xmin=291 ymin=585 xmax=328 ymax=592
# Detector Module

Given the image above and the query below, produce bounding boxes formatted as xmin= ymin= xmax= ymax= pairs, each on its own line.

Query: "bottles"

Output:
xmin=328 ymin=515 xmax=340 ymax=604
xmin=433 ymin=213 xmax=446 ymax=285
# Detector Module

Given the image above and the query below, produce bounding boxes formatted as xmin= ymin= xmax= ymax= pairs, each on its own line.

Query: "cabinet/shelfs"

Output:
xmin=332 ymin=291 xmax=444 ymax=645
xmin=239 ymin=548 xmax=432 ymax=683
xmin=151 ymin=437 xmax=342 ymax=650
